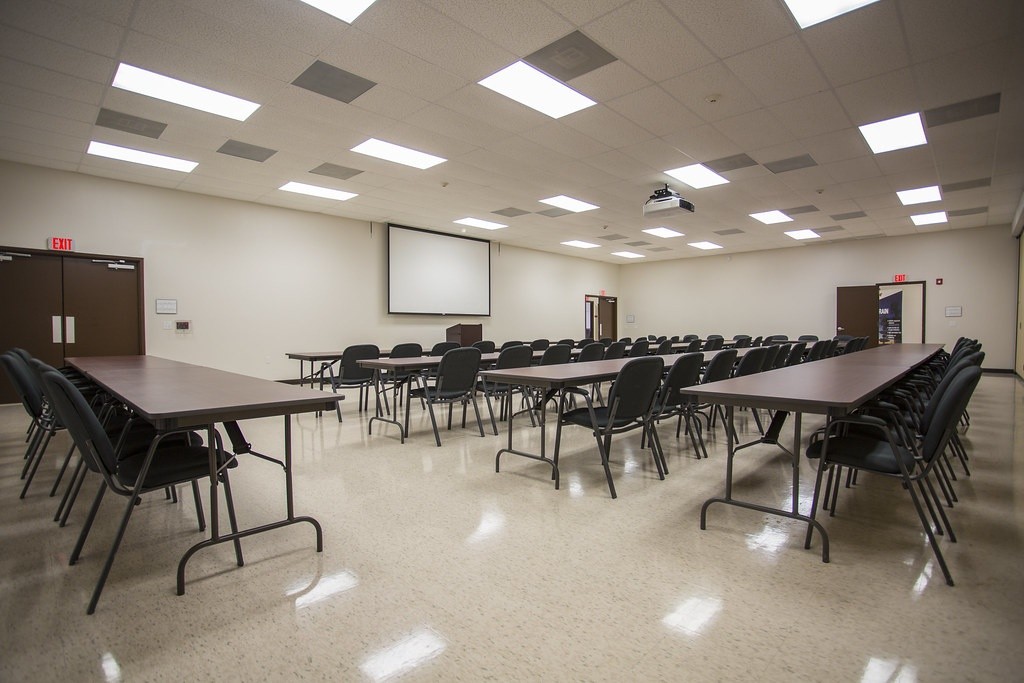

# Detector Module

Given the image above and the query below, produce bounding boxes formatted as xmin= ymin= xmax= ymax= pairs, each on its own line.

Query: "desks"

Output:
xmin=680 ymin=343 xmax=946 ymax=564
xmin=88 ymin=364 xmax=344 ymax=597
xmin=63 ymin=352 xmax=204 ymax=479
xmin=290 ymin=342 xmax=849 ymax=490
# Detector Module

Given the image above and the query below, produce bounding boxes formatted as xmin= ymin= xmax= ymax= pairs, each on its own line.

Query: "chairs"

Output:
xmin=0 ymin=347 xmax=154 ymax=519
xmin=37 ymin=362 xmax=205 ymax=526
xmin=406 ymin=346 xmax=483 ymax=447
xmin=364 ymin=345 xmax=421 ymax=414
xmin=410 ymin=343 xmax=461 ymax=409
xmin=321 ymin=346 xmax=384 ymax=422
xmin=42 ymin=372 xmax=245 ymax=614
xmin=803 ymin=364 xmax=982 ymax=585
xmin=472 ymin=333 xmax=987 ymax=528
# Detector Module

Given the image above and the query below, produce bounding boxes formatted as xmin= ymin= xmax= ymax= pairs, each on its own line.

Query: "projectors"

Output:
xmin=643 ymin=198 xmax=695 ymax=218
